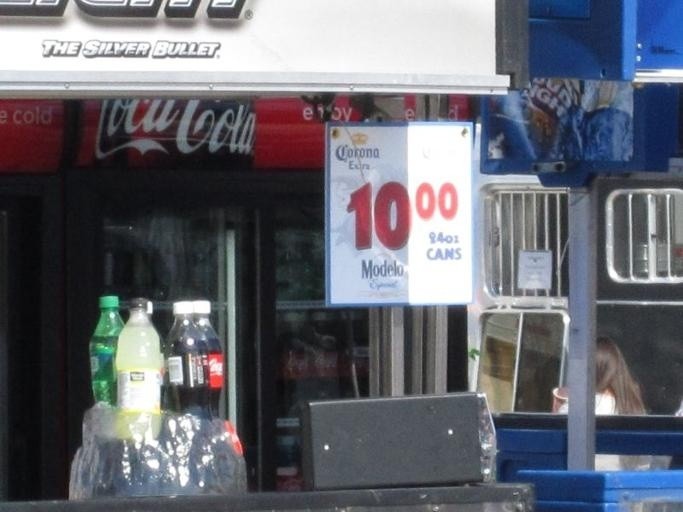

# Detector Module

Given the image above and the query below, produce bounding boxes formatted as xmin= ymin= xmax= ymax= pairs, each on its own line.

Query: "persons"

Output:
xmin=558 ymin=335 xmax=650 ymax=416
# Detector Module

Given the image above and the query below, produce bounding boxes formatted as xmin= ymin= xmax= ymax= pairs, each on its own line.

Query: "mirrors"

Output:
xmin=474 ymin=308 xmax=569 ymax=413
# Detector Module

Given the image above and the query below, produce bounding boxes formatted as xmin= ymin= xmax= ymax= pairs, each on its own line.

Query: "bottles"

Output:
xmin=87 ymin=296 xmax=225 ymax=420
